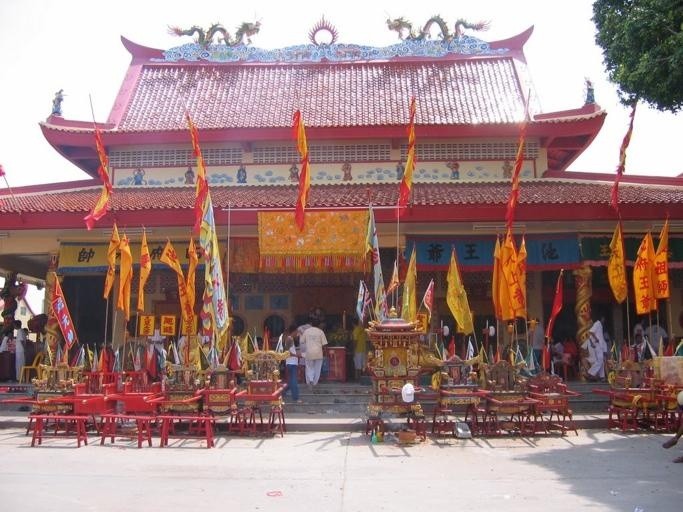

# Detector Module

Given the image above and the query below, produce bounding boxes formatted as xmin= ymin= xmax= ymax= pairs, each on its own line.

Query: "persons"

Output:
xmin=275 ymin=313 xmax=368 ymax=402
xmin=0 ymin=318 xmax=39 ymax=379
xmin=547 ymin=314 xmax=615 ymax=382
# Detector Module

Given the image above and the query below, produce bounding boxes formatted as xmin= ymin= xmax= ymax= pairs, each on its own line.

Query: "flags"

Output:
xmin=202 ymin=193 xmax=234 ymax=344
xmin=395 ymin=97 xmax=417 ymax=219
xmin=290 ymin=109 xmax=311 ymax=230
xmin=182 ymin=114 xmax=209 ymax=227
xmin=603 ymin=218 xmax=683 ymax=363
xmin=44 ymin=275 xmax=80 ymax=347
xmin=610 ymin=103 xmax=635 ymax=227
xmin=505 ymin=109 xmax=528 ymax=227
xmin=100 ymin=225 xmax=202 ymax=323
xmin=352 ymin=201 xmax=565 ymax=379
xmin=38 ymin=331 xmax=287 ymax=374
xmin=82 ymin=124 xmax=113 ymax=232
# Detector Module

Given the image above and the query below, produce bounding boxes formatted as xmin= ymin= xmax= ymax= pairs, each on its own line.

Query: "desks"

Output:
xmin=280 ymin=346 xmax=347 ymax=383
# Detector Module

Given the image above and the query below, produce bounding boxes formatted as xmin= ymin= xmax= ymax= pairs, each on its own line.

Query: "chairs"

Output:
xmin=593 ymin=354 xmax=682 ymax=448
xmin=25 ymin=338 xmax=289 ymax=449
xmin=360 ymin=338 xmax=582 ymax=440
